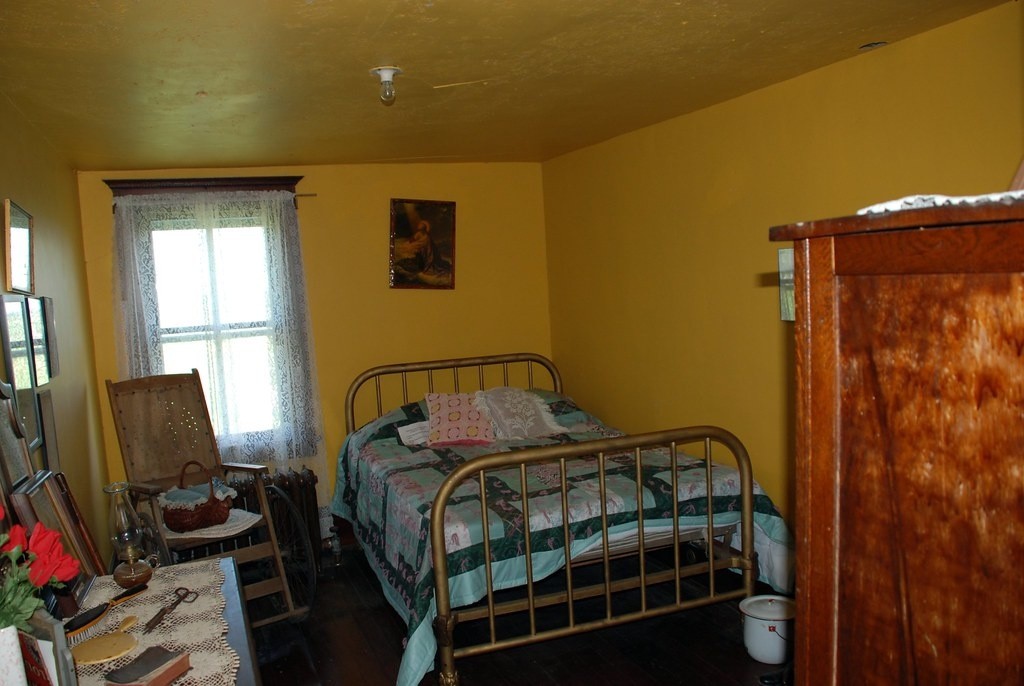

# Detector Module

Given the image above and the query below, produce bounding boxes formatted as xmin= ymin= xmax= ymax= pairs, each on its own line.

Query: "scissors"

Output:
xmin=165 ymin=587 xmax=199 ymax=614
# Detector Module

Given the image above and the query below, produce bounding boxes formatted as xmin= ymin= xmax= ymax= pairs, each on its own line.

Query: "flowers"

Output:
xmin=0 ymin=503 xmax=81 ymax=634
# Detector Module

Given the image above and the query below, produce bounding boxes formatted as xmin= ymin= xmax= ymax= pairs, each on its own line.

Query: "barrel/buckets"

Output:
xmin=738 ymin=595 xmax=794 ymax=664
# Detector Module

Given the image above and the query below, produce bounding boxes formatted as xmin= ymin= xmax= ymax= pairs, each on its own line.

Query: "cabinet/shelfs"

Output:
xmin=769 ymin=200 xmax=1024 ymax=685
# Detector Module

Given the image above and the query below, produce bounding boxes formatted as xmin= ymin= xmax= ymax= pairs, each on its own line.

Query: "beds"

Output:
xmin=331 ymin=353 xmax=797 ymax=686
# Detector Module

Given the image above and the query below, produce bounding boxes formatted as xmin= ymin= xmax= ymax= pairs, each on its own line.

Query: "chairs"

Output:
xmin=104 ymin=368 xmax=310 ymax=628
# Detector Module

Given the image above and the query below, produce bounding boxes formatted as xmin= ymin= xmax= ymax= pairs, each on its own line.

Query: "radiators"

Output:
xmin=175 ymin=465 xmax=324 ymax=576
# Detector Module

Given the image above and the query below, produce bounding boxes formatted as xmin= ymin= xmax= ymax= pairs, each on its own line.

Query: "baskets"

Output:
xmin=163 ymin=461 xmax=229 ymax=533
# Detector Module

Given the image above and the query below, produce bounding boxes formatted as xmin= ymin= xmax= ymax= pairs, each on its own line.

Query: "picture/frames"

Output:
xmin=4 ymin=199 xmax=35 ymax=296
xmin=37 ymin=389 xmax=61 ymax=475
xmin=0 ymin=294 xmax=44 ymax=453
xmin=390 ymin=197 xmax=456 ymax=291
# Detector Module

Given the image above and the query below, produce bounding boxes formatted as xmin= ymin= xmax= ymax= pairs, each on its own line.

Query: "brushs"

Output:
xmin=63 ymin=583 xmax=149 ymax=646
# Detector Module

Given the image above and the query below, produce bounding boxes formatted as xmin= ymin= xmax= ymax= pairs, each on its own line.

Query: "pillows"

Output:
xmin=472 ymin=388 xmax=569 ymax=440
xmin=423 ymin=393 xmax=496 ymax=446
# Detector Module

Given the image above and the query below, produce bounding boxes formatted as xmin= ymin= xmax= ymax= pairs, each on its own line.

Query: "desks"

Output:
xmin=63 ymin=557 xmax=261 ymax=686
